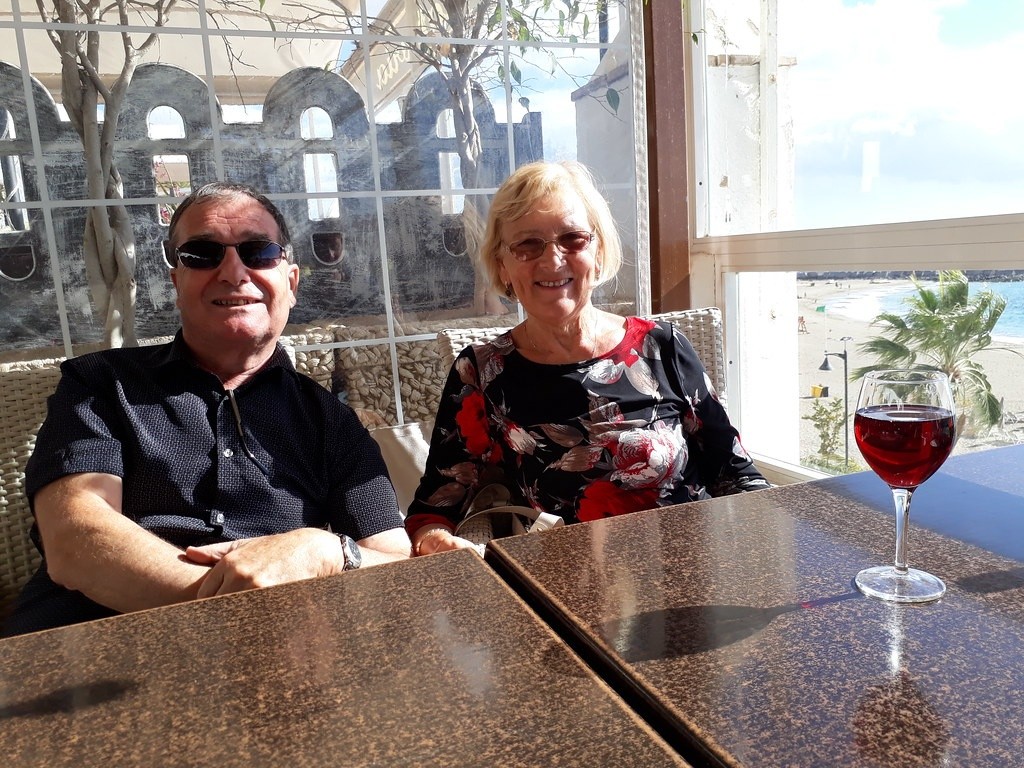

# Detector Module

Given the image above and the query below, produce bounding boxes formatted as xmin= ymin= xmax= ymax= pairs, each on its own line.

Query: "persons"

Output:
xmin=800 ymin=316 xmax=806 ymax=331
xmin=403 ymin=160 xmax=774 ymax=556
xmin=289 ymin=196 xmax=510 ymax=324
xmin=0 ymin=181 xmax=418 ymax=642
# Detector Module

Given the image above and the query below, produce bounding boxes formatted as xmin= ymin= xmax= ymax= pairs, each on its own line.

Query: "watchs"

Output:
xmin=334 ymin=533 xmax=362 ymax=572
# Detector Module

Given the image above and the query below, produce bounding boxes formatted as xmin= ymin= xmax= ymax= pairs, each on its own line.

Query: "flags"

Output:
xmin=816 ymin=306 xmax=825 ymax=312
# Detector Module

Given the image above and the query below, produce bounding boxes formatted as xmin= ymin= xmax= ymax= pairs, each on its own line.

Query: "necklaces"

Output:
xmin=524 ymin=312 xmax=598 ymax=364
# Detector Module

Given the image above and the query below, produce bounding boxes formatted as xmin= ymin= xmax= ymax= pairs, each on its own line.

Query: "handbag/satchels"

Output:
xmin=453 ymin=501 xmax=566 ymax=561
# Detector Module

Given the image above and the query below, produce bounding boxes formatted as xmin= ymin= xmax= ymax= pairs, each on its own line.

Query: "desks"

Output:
xmin=0 ymin=545 xmax=688 ymax=768
xmin=486 ymin=444 xmax=1023 ymax=768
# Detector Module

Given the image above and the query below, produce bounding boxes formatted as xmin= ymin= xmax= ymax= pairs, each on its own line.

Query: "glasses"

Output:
xmin=501 ymin=227 xmax=596 ymax=262
xmin=174 ymin=238 xmax=291 ymax=269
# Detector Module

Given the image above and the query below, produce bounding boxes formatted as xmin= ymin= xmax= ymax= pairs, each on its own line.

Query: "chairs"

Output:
xmin=437 ymin=304 xmax=729 ymax=551
xmin=0 ymin=341 xmax=298 ymax=628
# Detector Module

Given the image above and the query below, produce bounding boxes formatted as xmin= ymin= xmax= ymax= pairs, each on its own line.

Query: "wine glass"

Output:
xmin=854 ymin=369 xmax=956 ymax=601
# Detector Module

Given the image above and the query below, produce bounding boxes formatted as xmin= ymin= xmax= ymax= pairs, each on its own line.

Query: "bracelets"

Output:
xmin=416 ymin=528 xmax=450 ymax=556
